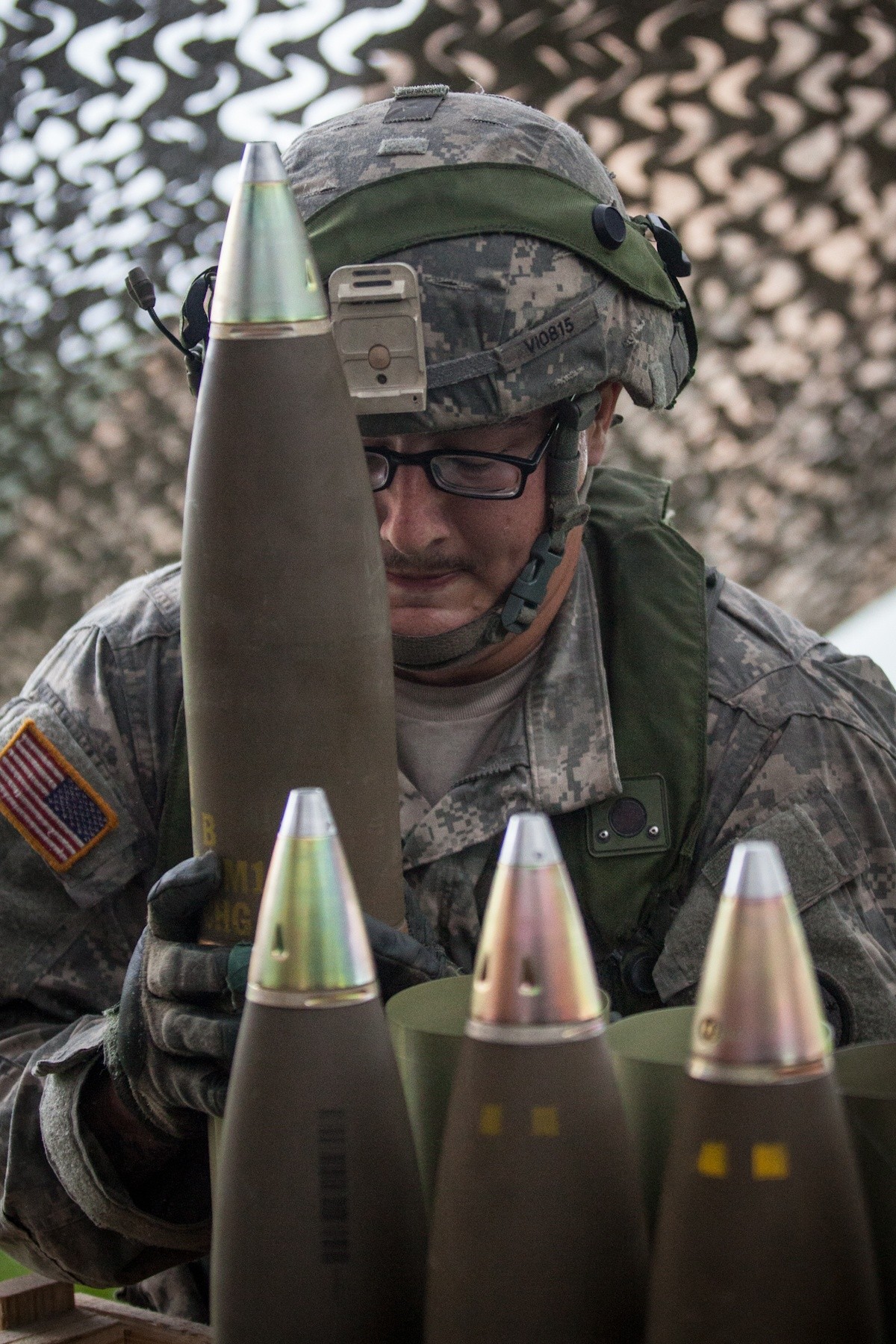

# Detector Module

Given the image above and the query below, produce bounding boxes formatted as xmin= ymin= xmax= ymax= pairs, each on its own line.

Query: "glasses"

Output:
xmin=364 ymin=392 xmax=577 ymax=501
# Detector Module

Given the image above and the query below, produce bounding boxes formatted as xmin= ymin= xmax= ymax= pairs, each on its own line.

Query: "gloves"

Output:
xmin=360 ymin=873 xmax=454 ymax=1003
xmin=118 ymin=849 xmax=256 ymax=1139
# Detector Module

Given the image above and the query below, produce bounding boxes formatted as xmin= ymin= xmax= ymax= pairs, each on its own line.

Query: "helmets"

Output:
xmin=179 ymin=83 xmax=698 ymax=437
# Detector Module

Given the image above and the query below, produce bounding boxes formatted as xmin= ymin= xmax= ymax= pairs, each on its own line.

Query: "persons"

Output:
xmin=0 ymin=83 xmax=896 ymax=1326
